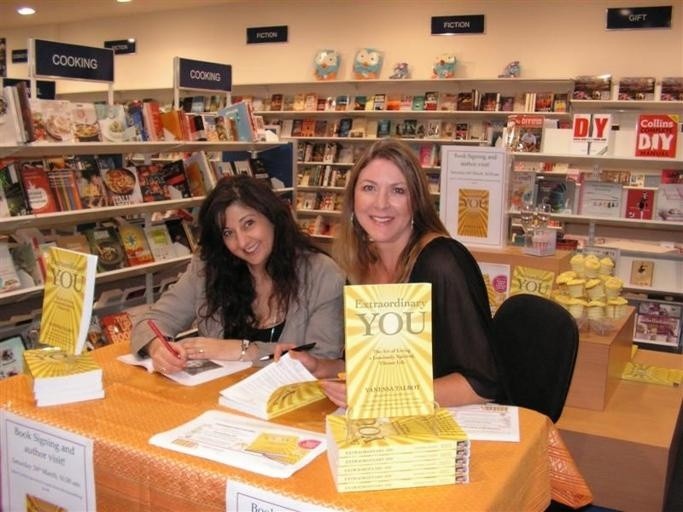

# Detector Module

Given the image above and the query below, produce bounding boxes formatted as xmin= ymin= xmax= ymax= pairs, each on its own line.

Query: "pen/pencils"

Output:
xmin=148 ymin=320 xmax=186 ymax=369
xmin=259 ymin=341 xmax=317 ymax=361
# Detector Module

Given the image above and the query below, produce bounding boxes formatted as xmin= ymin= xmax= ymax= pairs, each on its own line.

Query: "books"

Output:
xmin=217 ymin=350 xmax=347 ymax=423
xmin=18 ymin=346 xmax=108 ymax=408
xmin=342 ymin=282 xmax=437 ymax=420
xmin=322 ymin=405 xmax=471 ymax=494
xmin=117 ymin=352 xmax=256 ymax=387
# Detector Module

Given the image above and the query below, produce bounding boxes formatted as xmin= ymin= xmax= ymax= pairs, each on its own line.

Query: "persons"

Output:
xmin=271 ymin=136 xmax=505 ymax=412
xmin=129 ymin=171 xmax=349 ymax=377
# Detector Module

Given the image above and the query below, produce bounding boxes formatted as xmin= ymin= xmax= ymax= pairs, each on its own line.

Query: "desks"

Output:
xmin=0 ymin=339 xmax=593 ymax=512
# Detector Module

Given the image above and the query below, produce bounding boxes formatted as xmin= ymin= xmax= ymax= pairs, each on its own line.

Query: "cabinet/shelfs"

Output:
xmin=0 ymin=78 xmax=683 ymax=379
